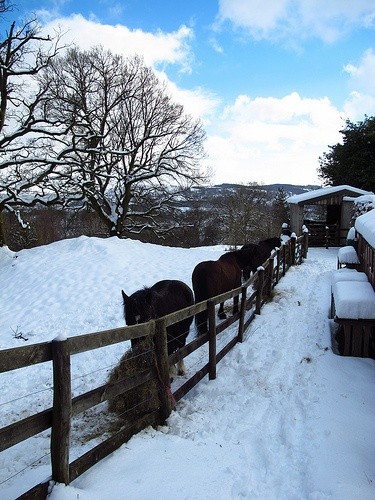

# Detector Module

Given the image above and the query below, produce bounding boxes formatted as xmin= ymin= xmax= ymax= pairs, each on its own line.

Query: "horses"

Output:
xmin=237 ymin=233 xmax=285 ymax=286
xmin=116 ymin=277 xmax=199 ymax=384
xmin=191 ymin=247 xmax=255 ymax=333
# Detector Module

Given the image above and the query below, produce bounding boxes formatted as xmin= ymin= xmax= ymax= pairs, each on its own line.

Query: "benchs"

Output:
xmin=331 ymin=246 xmax=375 ymax=357
